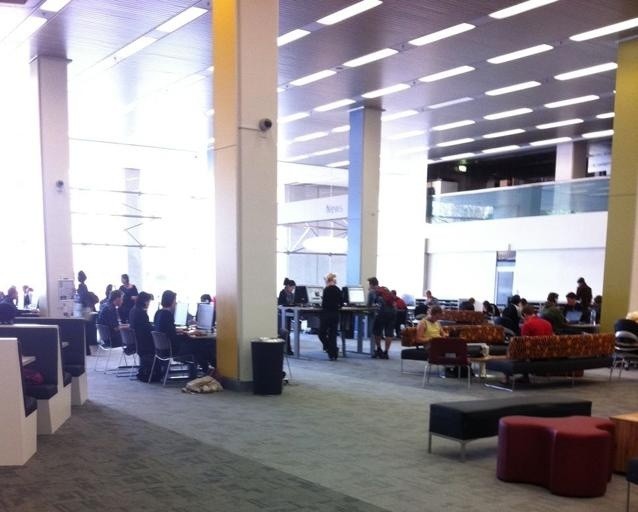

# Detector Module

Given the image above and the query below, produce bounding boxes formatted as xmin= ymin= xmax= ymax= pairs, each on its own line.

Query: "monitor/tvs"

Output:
xmin=195 ymin=303 xmax=215 ymax=330
xmin=173 ymin=302 xmax=190 ymax=325
xmin=566 ymin=312 xmax=582 ymax=321
xmin=146 ymin=299 xmax=159 ymax=322
xmin=347 ymin=287 xmax=365 ymax=305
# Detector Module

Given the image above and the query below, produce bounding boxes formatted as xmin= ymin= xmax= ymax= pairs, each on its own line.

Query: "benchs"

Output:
xmin=427 ymin=391 xmax=637 ymax=511
xmin=0 ymin=314 xmax=90 ymax=469
xmin=428 ymin=310 xmax=489 ymax=325
xmin=479 ymin=333 xmax=614 ymax=391
xmin=438 ymin=324 xmax=510 ymax=355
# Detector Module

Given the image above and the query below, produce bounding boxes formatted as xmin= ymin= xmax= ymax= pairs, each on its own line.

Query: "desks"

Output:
xmin=568 ymin=324 xmax=602 ymax=332
xmin=278 ymin=304 xmax=378 ymax=358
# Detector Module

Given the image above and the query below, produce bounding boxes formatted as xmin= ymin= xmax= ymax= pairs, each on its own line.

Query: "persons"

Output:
xmin=458 ymin=275 xmax=637 ymax=345
xmin=278 ymin=275 xmax=297 ymax=354
xmin=415 ymin=306 xmax=474 ymax=379
xmin=424 ymin=287 xmax=438 ymax=307
xmin=390 ymin=288 xmax=407 ymax=340
xmin=364 ymin=276 xmax=390 ymax=361
xmin=320 ymin=273 xmax=342 ymax=361
xmin=0 ymin=269 xmax=219 ymax=382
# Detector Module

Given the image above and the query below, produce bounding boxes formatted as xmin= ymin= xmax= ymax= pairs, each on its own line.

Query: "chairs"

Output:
xmin=423 ymin=337 xmax=473 ymax=390
xmin=614 ymin=318 xmax=636 ymax=335
xmin=279 ymin=328 xmax=293 ymax=379
xmin=608 ymin=331 xmax=637 ymax=383
xmin=401 ymin=327 xmax=441 ymax=377
xmin=93 ymin=299 xmax=215 ymax=387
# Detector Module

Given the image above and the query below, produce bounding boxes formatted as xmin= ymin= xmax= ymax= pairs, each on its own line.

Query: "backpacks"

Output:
xmin=368 ymin=286 xmax=393 ymax=305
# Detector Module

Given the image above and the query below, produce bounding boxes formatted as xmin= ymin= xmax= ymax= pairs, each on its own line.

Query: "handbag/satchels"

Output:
xmin=181 ymin=375 xmax=223 ymax=394
xmin=446 ymin=366 xmax=473 ymax=378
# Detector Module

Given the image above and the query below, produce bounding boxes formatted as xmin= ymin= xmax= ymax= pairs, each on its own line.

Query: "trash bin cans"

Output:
xmin=250 ymin=335 xmax=287 ymax=395
